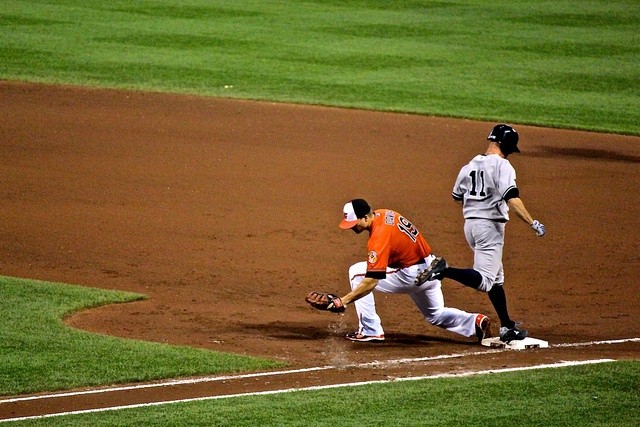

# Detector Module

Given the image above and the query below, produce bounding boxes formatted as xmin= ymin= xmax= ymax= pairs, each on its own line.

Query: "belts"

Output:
xmin=416 ymin=253 xmax=432 ymax=264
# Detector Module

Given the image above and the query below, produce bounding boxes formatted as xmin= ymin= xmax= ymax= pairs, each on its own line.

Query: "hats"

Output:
xmin=338 ymin=199 xmax=371 ymax=230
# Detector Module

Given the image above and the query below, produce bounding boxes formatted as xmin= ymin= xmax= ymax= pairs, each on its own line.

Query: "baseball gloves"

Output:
xmin=305 ymin=290 xmax=346 ymax=313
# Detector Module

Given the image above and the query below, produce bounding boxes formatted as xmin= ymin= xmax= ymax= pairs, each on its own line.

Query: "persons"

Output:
xmin=327 ymin=198 xmax=492 ymax=345
xmin=451 ymin=124 xmax=545 ymax=341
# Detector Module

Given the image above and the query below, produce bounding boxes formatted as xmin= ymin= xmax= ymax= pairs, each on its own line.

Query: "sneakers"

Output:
xmin=499 ymin=326 xmax=528 ymax=342
xmin=346 ymin=331 xmax=385 ymax=341
xmin=475 ymin=314 xmax=492 ymax=345
xmin=414 ymin=257 xmax=447 ymax=286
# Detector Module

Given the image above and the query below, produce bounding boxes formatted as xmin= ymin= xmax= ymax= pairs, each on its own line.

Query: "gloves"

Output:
xmin=531 ymin=220 xmax=546 ymax=237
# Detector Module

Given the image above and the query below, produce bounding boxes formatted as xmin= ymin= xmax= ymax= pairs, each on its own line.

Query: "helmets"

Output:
xmin=487 ymin=124 xmax=520 ymax=152
